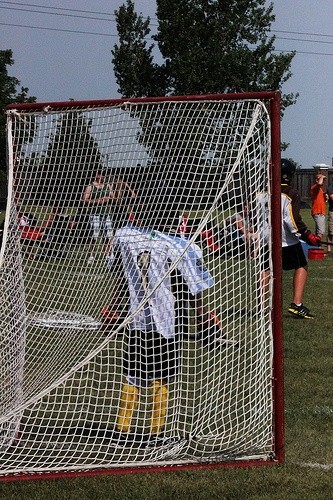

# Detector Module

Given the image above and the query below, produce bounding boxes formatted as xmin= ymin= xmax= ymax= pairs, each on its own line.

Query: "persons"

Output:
xmin=74 ymin=169 xmax=333 ymax=442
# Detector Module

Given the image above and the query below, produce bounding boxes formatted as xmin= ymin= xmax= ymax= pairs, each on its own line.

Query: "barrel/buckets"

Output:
xmin=308 ymin=249 xmax=324 ymax=260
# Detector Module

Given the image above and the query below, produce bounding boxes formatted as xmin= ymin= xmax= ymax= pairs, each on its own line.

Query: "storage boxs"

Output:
xmin=308 ymin=248 xmax=324 ymax=261
